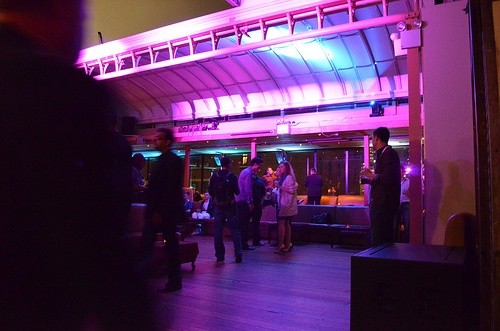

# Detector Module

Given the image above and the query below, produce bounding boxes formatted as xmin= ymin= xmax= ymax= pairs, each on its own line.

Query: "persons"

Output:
xmin=0 ymin=0 xmax=324 ymax=331
xmin=361 ymin=127 xmax=425 ymax=247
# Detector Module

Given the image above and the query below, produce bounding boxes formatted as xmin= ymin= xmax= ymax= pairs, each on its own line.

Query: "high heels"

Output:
xmin=274 ymin=244 xmax=285 ymax=255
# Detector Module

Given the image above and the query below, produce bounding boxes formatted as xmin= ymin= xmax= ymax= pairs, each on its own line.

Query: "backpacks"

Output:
xmin=213 ymin=171 xmax=236 ymax=207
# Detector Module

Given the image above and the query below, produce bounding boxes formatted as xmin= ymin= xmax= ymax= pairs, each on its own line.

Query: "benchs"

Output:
xmin=195 ymin=195 xmax=372 ymax=250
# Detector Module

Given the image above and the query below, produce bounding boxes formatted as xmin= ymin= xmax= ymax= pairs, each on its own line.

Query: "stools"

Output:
xmin=153 ymin=240 xmax=199 ymax=271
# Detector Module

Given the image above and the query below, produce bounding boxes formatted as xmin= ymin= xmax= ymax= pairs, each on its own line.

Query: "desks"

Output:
xmin=126 ymin=203 xmax=184 ymax=243
xmin=351 ymin=242 xmax=483 ymax=331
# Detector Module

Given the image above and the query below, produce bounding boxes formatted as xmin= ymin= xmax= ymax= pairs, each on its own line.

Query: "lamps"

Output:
xmin=369 ymin=100 xmax=384 ymax=116
xmin=390 ymin=33 xmax=407 ymax=57
xmin=396 ymin=19 xmax=423 ymax=49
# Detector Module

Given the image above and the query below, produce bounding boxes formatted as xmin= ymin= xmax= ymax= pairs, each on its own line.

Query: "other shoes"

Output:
xmin=253 ymin=240 xmax=265 ymax=246
xmin=236 ymin=257 xmax=242 ymax=263
xmin=242 ymin=244 xmax=256 ymax=250
xmin=164 ymin=282 xmax=182 ymax=292
xmin=285 ymin=243 xmax=293 ymax=252
xmin=216 ymin=261 xmax=224 ymax=263
